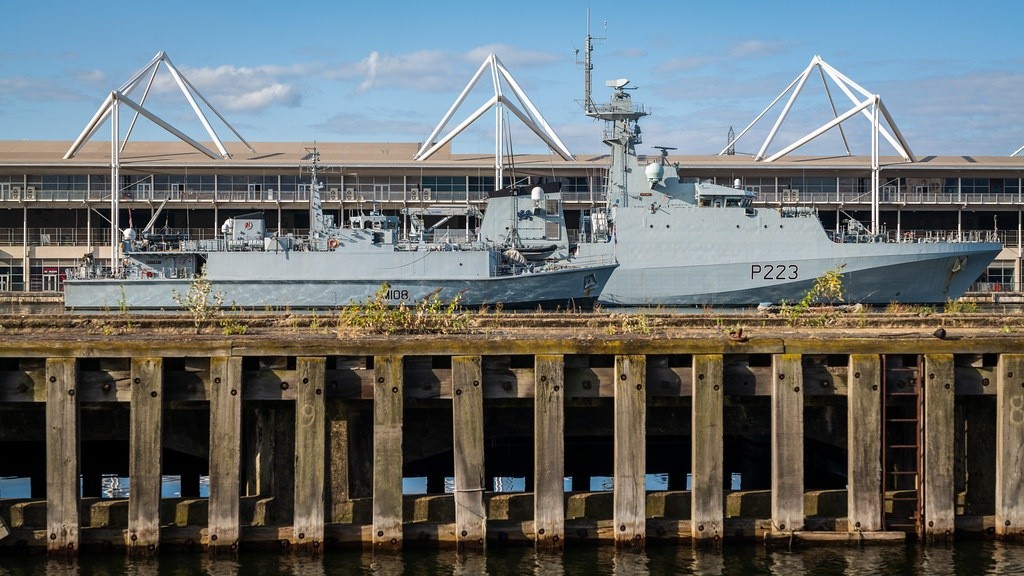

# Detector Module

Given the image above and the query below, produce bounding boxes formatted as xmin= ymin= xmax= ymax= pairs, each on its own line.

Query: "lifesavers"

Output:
xmin=328 ymin=239 xmax=337 ymax=248
xmin=244 ymin=222 xmax=253 ymax=230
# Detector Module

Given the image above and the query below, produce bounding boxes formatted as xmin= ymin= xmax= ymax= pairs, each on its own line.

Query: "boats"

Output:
xmin=60 ymin=139 xmax=624 ymax=319
xmin=546 ymin=3 xmax=1004 ymax=313
